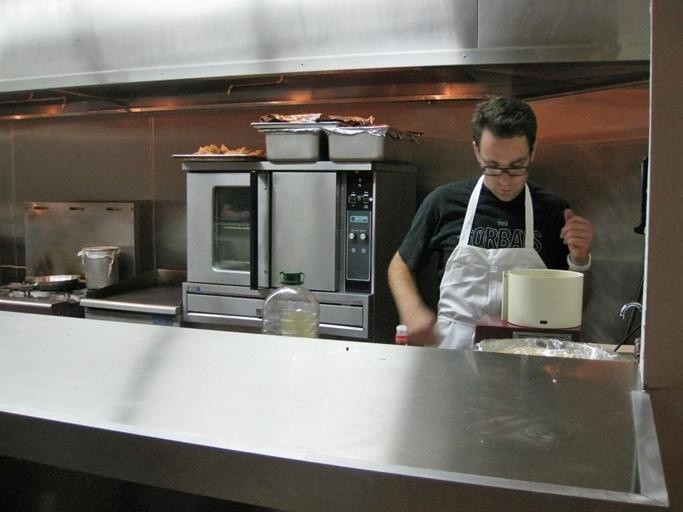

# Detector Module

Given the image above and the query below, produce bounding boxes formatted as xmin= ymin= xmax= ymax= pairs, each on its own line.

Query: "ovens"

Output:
xmin=182 ymin=158 xmax=418 ymax=343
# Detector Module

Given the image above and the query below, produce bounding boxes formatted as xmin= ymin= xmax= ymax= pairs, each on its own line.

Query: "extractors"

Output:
xmin=1 ymin=2 xmax=649 ymax=124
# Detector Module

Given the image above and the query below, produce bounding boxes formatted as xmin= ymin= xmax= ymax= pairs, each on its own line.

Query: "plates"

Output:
xmin=249 ymin=119 xmax=367 ymax=129
xmin=171 ymin=154 xmax=265 ymax=162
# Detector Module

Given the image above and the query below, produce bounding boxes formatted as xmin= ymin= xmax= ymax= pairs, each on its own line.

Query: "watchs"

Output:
xmin=564 ymin=252 xmax=592 ymax=271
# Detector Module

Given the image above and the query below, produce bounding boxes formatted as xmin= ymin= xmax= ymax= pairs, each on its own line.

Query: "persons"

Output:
xmin=387 ymin=96 xmax=595 ymax=351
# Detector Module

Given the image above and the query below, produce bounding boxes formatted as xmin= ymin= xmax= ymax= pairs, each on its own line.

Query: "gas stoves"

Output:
xmin=0 ymin=285 xmax=84 ymax=313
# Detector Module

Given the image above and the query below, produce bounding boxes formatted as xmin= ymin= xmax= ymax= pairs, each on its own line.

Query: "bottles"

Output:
xmin=261 ymin=272 xmax=319 ymax=338
xmin=396 ymin=325 xmax=407 ymax=345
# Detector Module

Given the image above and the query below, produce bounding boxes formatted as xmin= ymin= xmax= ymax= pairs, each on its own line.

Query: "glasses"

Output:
xmin=475 ymin=145 xmax=532 ymax=177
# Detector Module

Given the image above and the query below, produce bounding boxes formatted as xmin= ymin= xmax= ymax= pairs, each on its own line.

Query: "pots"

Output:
xmin=24 ymin=274 xmax=82 ymax=291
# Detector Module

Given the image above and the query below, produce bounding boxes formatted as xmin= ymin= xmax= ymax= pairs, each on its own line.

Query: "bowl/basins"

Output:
xmin=261 ymin=130 xmax=328 ymax=158
xmin=331 ymin=127 xmax=419 ymax=161
